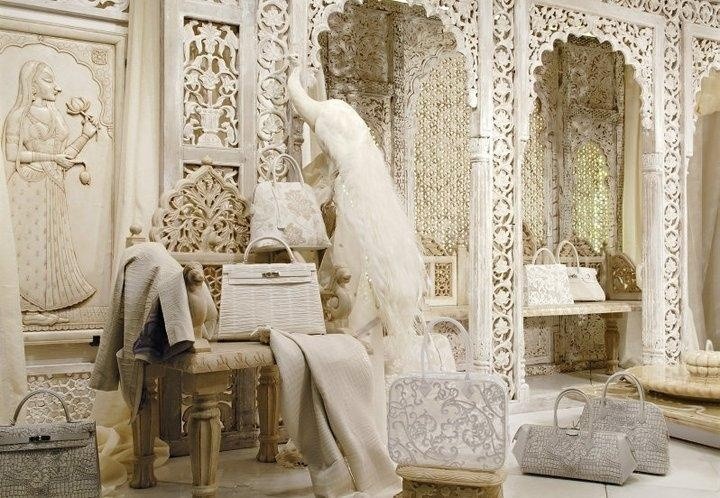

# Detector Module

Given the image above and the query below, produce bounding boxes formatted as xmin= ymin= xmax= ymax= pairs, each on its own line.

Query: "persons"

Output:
xmin=2 ymin=56 xmax=103 ymax=331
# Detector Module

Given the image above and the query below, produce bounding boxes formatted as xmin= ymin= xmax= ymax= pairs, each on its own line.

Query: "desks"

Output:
xmin=428 ymin=299 xmax=633 ymax=375
xmin=117 ymin=338 xmax=376 ymax=497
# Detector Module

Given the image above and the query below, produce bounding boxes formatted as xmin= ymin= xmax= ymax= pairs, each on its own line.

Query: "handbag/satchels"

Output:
xmin=218 ymin=237 xmax=326 ymax=340
xmin=0 ymin=390 xmax=100 ymax=498
xmin=523 ymin=241 xmax=606 ymax=307
xmin=389 ymin=317 xmax=507 ymax=470
xmin=512 ymin=373 xmax=670 ymax=486
xmin=250 ymin=154 xmax=331 ymax=252
